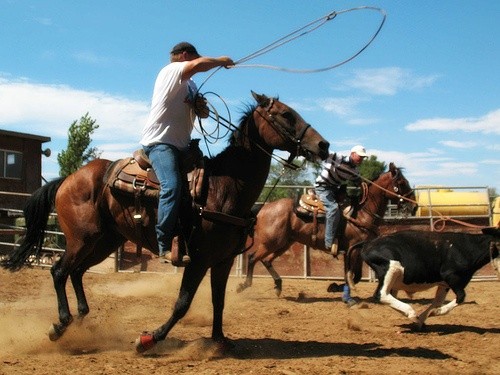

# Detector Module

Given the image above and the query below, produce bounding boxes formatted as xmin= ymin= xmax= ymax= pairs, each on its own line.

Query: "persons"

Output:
xmin=138 ymin=40 xmax=236 ymax=264
xmin=313 ymin=145 xmax=368 ymax=255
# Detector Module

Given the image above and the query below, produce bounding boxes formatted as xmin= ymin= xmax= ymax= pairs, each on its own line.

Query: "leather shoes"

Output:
xmin=160 ymin=250 xmax=192 ymax=267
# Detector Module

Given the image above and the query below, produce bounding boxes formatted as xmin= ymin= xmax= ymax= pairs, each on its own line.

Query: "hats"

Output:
xmin=171 ymin=42 xmax=202 ymax=58
xmin=350 ymin=145 xmax=368 ymax=158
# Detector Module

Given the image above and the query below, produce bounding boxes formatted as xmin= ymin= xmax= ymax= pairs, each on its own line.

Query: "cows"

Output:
xmin=345 ymin=228 xmax=500 ymax=331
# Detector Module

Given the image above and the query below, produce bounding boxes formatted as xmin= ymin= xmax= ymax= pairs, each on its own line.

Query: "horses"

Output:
xmin=0 ymin=89 xmax=330 ymax=353
xmin=235 ymin=161 xmax=418 ymax=298
